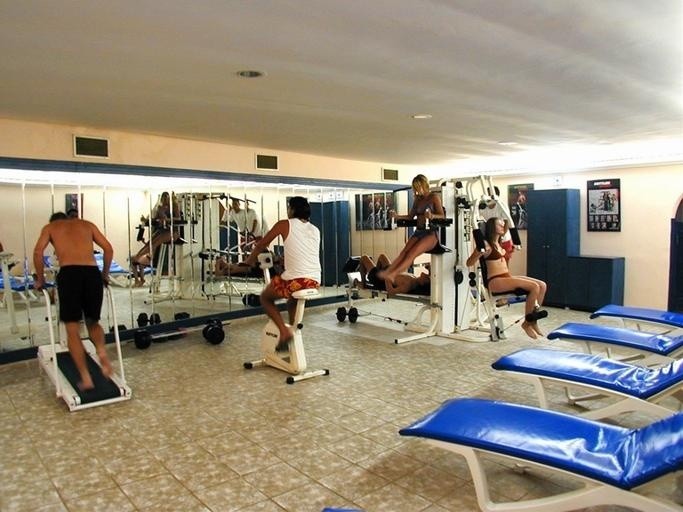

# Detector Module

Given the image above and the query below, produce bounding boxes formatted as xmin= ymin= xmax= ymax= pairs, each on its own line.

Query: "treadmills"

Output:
xmin=36 ymin=255 xmax=133 ymax=411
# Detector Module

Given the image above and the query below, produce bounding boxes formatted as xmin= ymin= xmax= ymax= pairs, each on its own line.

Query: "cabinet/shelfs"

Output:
xmin=310 ymin=199 xmax=349 ymax=288
xmin=568 ymin=254 xmax=624 ymax=312
xmin=527 ymin=189 xmax=580 ymax=311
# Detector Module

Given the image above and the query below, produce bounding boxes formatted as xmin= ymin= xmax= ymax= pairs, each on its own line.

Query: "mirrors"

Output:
xmin=0 ymin=157 xmax=415 ymax=366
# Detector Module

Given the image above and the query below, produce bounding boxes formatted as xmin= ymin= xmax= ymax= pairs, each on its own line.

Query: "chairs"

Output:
xmin=547 ymin=322 xmax=683 ymax=368
xmin=491 ymin=348 xmax=683 ymax=412
xmin=589 ymin=305 xmax=683 ymax=335
xmin=0 ymin=253 xmax=158 ymax=307
xmin=322 ymin=396 xmax=683 ymax=512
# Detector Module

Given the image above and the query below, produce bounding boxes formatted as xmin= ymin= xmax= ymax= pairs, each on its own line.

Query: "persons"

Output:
xmin=33 ymin=212 xmax=114 ymax=392
xmin=242 ymin=197 xmax=322 ymax=351
xmin=470 ymin=186 xmax=508 ymax=223
xmin=130 ymin=192 xmax=181 ymax=266
xmin=215 ymin=253 xmax=285 ymax=279
xmin=221 ymin=199 xmax=259 ymax=249
xmin=466 ymin=217 xmax=548 ymax=340
xmin=356 ymin=254 xmax=431 ymax=295
xmin=515 ymin=190 xmax=527 ymax=218
xmin=0 ymin=243 xmax=27 ymax=284
xmin=375 ymin=174 xmax=445 ymax=285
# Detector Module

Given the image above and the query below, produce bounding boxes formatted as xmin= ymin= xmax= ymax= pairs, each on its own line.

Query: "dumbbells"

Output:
xmin=336 ymin=307 xmax=358 ymax=323
xmin=138 ymin=313 xmax=161 ymax=326
xmin=203 ymin=326 xmax=223 ymax=344
xmin=242 ymin=293 xmax=260 ymax=305
xmin=137 ymin=331 xmax=152 ymax=350
xmin=173 ymin=311 xmax=190 ymax=319
